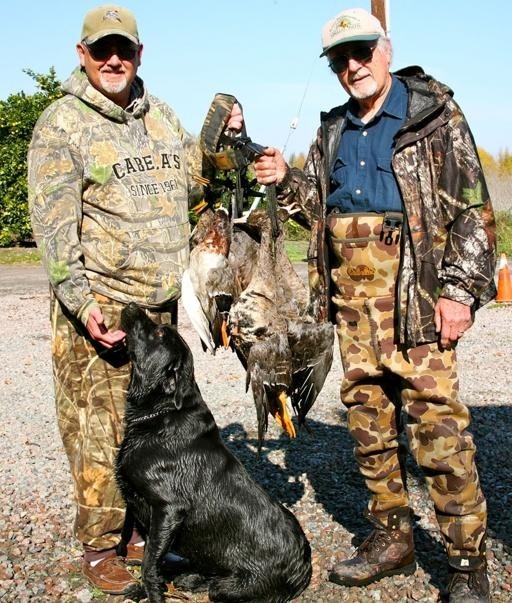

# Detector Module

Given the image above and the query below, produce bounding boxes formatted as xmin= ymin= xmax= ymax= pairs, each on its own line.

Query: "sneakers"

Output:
xmin=121 ymin=542 xmax=147 ymax=565
xmin=83 ymin=552 xmax=140 ymax=594
xmin=445 ymin=554 xmax=492 ymax=602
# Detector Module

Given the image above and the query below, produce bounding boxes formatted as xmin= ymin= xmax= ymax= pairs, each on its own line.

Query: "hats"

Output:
xmin=319 ymin=7 xmax=386 ymax=58
xmin=80 ymin=4 xmax=140 ymax=46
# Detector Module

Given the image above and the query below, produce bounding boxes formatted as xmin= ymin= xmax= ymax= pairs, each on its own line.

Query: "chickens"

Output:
xmin=181 ymin=170 xmax=335 ymax=460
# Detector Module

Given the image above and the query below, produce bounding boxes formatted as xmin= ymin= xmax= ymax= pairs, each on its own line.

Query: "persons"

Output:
xmin=252 ymin=1 xmax=502 ymax=602
xmin=26 ymin=1 xmax=244 ymax=595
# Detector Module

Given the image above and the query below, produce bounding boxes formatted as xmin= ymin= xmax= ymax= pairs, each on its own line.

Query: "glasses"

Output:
xmin=88 ymin=46 xmax=137 ymax=60
xmin=328 ymin=45 xmax=376 ymax=73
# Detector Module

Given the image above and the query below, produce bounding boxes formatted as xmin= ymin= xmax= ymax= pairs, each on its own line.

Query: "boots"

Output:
xmin=330 ymin=506 xmax=417 ymax=587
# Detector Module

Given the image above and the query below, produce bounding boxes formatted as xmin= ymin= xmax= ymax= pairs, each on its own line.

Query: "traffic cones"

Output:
xmin=487 ymin=249 xmax=512 ymax=309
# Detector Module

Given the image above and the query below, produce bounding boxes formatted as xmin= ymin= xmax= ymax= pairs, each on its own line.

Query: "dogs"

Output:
xmin=113 ymin=302 xmax=312 ymax=603
